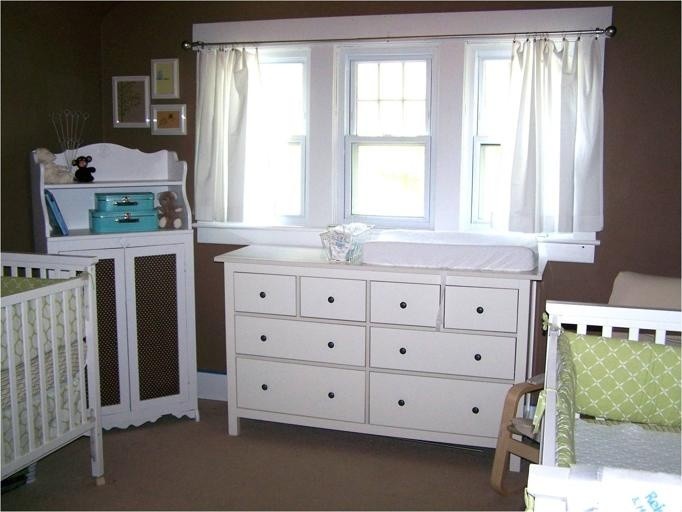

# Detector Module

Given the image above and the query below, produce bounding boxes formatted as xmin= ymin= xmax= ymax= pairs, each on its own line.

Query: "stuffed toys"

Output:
xmin=70 ymin=154 xmax=95 ymax=182
xmin=32 ymin=146 xmax=74 ymax=185
xmin=154 ymin=189 xmax=185 ymax=230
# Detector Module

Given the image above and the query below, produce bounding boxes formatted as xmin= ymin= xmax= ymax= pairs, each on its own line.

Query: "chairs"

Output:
xmin=488 ymin=271 xmax=680 ymax=496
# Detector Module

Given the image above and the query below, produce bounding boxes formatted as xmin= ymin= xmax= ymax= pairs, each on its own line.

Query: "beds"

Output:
xmin=0 ymin=251 xmax=106 ymax=482
xmin=527 ymin=297 xmax=682 ymax=511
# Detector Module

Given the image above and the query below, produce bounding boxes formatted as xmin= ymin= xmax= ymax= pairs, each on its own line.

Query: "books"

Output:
xmin=43 ymin=190 xmax=69 ymax=236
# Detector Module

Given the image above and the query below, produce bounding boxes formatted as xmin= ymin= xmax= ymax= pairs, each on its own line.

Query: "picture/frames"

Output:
xmin=111 ymin=58 xmax=187 ymax=137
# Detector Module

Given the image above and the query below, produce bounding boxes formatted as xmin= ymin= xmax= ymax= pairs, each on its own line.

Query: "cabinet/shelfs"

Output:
xmin=212 ymin=245 xmax=547 ymax=472
xmin=30 ymin=143 xmax=202 ymax=437
